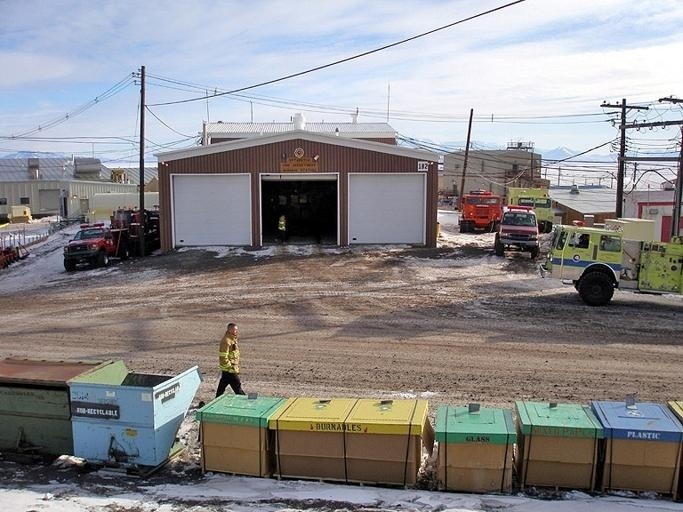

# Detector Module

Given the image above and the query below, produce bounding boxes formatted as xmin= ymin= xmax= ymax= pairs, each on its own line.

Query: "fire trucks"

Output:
xmin=504 ymin=186 xmax=554 ymax=233
xmin=539 ymin=214 xmax=683 ymax=308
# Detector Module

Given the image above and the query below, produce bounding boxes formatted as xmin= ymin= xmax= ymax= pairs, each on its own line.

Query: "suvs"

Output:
xmin=494 ymin=204 xmax=542 ymax=257
xmin=62 ymin=205 xmax=159 ymax=272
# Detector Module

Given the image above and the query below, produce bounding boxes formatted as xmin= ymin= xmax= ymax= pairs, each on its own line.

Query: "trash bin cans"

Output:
xmin=66 ymin=360 xmax=200 ymax=466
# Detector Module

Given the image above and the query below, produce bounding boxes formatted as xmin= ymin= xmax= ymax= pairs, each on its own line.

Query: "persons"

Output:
xmin=214 ymin=323 xmax=245 ymax=398
xmin=275 ymin=214 xmax=288 ymax=244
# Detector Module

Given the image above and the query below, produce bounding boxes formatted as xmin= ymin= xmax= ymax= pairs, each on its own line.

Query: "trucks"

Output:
xmin=455 ymin=189 xmax=502 ymax=234
